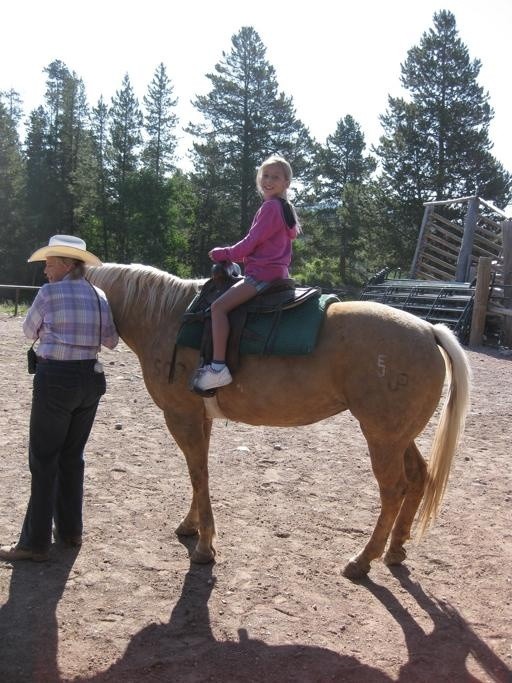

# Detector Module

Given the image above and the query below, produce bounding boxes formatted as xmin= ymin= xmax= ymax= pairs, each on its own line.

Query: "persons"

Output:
xmin=194 ymin=157 xmax=298 ymax=391
xmin=0 ymin=256 xmax=118 ymax=561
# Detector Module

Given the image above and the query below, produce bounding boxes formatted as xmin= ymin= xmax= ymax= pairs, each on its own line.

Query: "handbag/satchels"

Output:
xmin=27 ymin=348 xmax=37 ymax=374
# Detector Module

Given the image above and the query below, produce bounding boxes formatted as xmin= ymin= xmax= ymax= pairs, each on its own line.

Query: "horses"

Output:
xmin=83 ymin=260 xmax=472 ymax=581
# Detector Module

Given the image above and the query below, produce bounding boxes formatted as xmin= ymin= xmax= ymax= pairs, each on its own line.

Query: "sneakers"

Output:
xmin=0 ymin=545 xmax=49 ymax=562
xmin=50 ymin=526 xmax=83 ymax=545
xmin=191 ymin=365 xmax=233 ymax=392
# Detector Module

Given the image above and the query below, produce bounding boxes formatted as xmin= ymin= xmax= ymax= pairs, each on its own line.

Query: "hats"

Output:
xmin=27 ymin=234 xmax=103 ymax=267
